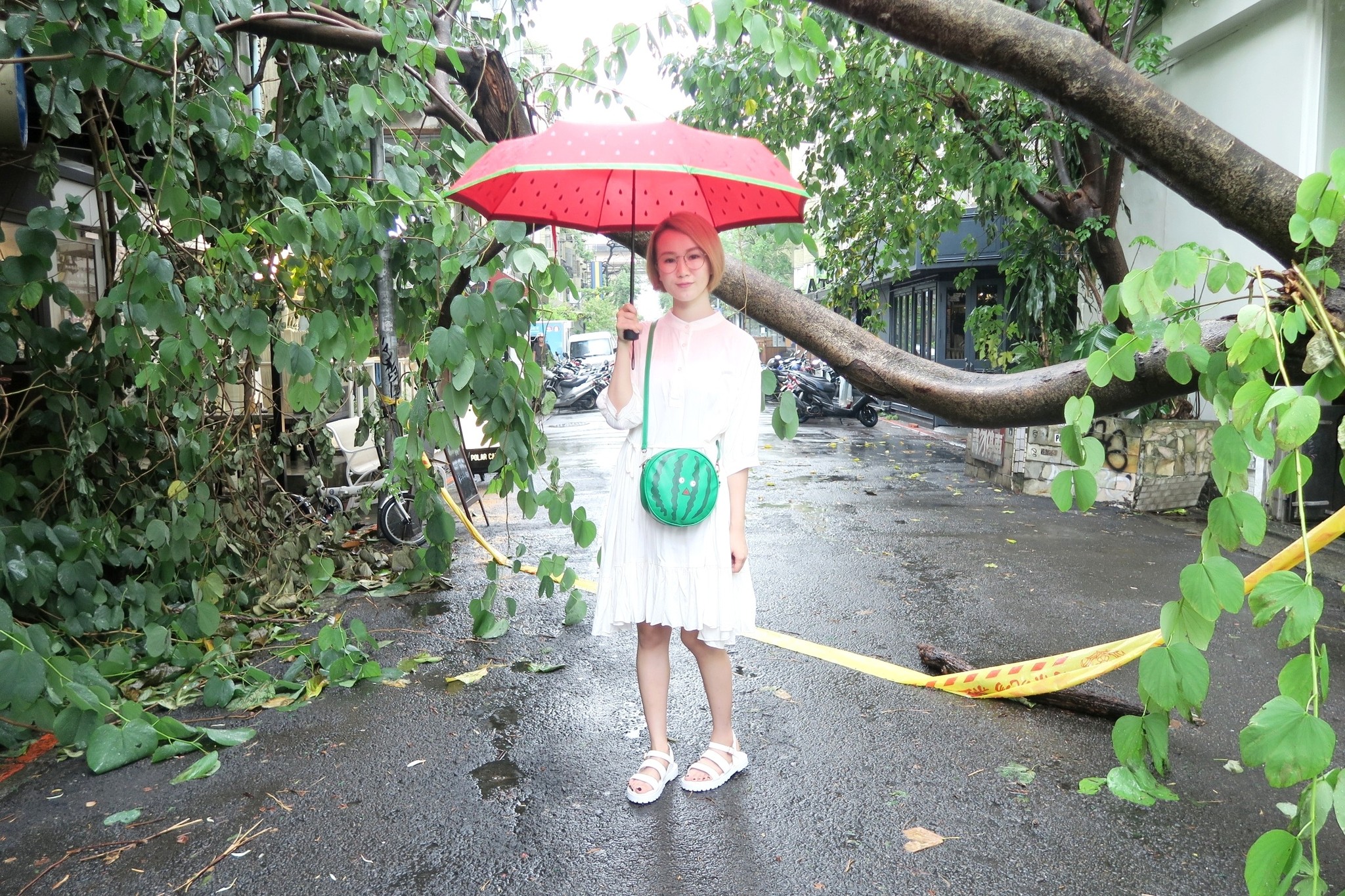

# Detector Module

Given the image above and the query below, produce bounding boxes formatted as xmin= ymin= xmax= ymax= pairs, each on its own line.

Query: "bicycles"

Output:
xmin=278 ymin=424 xmax=433 ymax=551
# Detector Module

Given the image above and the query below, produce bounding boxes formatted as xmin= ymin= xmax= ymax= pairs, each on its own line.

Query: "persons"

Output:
xmin=596 ymin=212 xmax=762 ymax=805
xmin=532 ymin=333 xmax=551 ymax=367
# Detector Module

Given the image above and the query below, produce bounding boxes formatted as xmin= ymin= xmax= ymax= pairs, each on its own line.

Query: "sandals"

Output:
xmin=681 ymin=728 xmax=749 ymax=791
xmin=627 ymin=743 xmax=679 ymax=802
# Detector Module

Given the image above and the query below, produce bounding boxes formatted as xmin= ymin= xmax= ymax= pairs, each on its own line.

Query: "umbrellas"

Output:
xmin=442 ymin=119 xmax=811 ymax=370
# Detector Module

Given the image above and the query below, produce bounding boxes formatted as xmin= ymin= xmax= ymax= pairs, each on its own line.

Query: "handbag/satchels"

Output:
xmin=641 ymin=447 xmax=720 ymax=528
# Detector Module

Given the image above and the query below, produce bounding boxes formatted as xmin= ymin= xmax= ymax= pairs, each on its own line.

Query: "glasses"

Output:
xmin=655 ymin=249 xmax=707 ymax=275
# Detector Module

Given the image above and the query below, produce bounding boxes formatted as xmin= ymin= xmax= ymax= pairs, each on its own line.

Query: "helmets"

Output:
xmin=537 ymin=332 xmax=543 ymax=342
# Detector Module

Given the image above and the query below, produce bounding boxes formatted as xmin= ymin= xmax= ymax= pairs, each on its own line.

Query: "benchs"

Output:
xmin=326 ymin=417 xmax=380 ymax=511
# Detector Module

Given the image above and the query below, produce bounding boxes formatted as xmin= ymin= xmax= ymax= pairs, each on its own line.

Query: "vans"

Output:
xmin=565 ymin=332 xmax=616 ymax=372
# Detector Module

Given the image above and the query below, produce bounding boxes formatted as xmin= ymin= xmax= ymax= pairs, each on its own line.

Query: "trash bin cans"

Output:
xmin=1287 ymin=405 xmax=1335 ymax=520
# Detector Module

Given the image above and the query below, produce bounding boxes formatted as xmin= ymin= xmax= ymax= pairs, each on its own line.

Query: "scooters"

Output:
xmin=759 ymin=349 xmax=880 ymax=427
xmin=531 ymin=352 xmax=616 ymax=415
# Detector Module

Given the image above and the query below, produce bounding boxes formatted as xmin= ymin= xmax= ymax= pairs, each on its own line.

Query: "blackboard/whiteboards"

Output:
xmin=427 ymin=400 xmax=481 ymax=509
